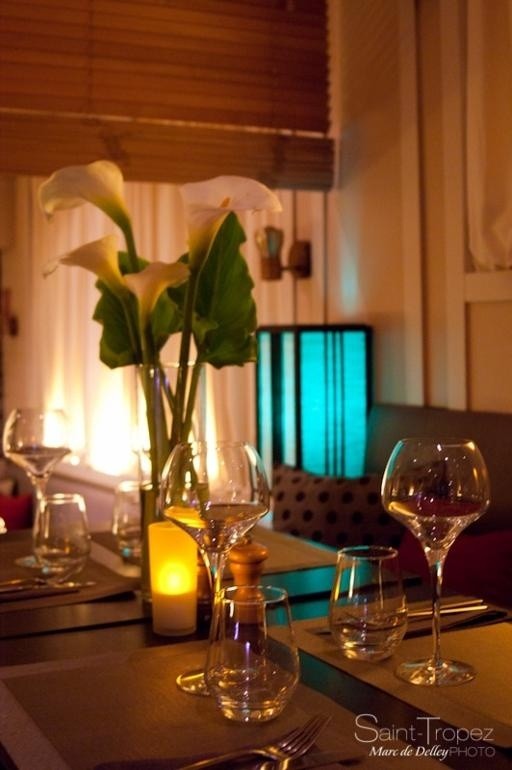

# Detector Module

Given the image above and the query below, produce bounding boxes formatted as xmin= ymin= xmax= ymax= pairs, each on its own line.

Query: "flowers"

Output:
xmin=37 ymin=157 xmax=284 ymax=520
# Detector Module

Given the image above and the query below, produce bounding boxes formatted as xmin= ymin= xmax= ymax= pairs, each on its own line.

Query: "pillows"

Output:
xmin=271 ymin=464 xmax=511 ymax=610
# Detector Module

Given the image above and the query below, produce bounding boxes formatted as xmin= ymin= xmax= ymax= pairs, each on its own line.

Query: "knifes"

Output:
xmin=1 ymin=581 xmax=97 ymax=602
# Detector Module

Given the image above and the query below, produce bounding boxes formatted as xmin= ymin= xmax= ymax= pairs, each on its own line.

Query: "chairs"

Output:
xmin=362 ymin=403 xmax=511 ymax=527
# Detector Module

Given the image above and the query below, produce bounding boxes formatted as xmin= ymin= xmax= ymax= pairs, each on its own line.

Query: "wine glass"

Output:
xmin=1 ymin=406 xmax=72 ymax=570
xmin=380 ymin=436 xmax=491 ymax=688
xmin=157 ymin=440 xmax=273 ymax=697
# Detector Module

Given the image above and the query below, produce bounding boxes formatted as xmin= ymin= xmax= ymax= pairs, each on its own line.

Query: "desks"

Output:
xmin=1 ymin=512 xmax=512 ymax=770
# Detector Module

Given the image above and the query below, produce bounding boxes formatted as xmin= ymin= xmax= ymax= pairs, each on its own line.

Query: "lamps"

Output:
xmin=252 ymin=226 xmax=314 ymax=283
xmin=148 ymin=524 xmax=200 ymax=635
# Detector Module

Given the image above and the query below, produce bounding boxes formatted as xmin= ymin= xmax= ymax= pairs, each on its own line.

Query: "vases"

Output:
xmin=135 ymin=362 xmax=209 ymax=639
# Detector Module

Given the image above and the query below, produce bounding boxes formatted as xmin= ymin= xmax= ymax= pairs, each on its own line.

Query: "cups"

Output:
xmin=32 ymin=493 xmax=91 ymax=589
xmin=206 ymin=583 xmax=301 ymax=726
xmin=112 ymin=483 xmax=145 ymax=562
xmin=328 ymin=544 xmax=408 ymax=663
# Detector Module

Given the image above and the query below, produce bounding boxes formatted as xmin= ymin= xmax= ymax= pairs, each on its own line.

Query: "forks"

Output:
xmin=0 ymin=566 xmax=84 ymax=585
xmin=168 ymin=712 xmax=333 ymax=770
